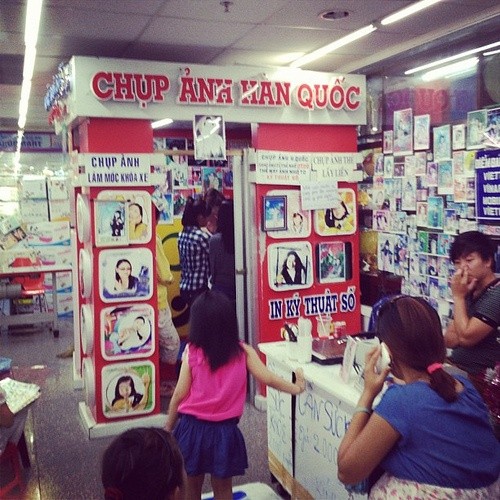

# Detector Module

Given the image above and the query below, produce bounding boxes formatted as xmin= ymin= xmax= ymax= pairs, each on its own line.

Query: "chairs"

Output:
xmin=9 ymin=258 xmax=51 ymax=330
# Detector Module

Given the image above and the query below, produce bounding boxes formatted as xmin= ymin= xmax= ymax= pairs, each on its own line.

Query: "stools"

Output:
xmin=0 ymin=434 xmax=31 ymax=500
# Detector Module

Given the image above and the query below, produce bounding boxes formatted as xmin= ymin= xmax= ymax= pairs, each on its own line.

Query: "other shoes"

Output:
xmin=158 ymin=382 xmax=176 ymax=397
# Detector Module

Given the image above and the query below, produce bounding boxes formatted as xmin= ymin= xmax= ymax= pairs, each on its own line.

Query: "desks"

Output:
xmin=1 ymin=264 xmax=73 ymax=338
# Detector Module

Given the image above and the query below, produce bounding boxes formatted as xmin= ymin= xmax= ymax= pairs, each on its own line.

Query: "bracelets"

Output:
xmin=355 ymin=407 xmax=370 ymax=417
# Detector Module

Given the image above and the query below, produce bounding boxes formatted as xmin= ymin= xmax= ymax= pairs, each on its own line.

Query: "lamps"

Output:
xmin=288 ymin=0 xmax=442 ymax=67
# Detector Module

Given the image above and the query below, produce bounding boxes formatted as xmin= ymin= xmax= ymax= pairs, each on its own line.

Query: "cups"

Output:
xmin=317 ymin=317 xmax=332 ymax=336
xmin=288 ymin=341 xmax=297 ymax=360
xmin=297 ymin=336 xmax=313 ymax=362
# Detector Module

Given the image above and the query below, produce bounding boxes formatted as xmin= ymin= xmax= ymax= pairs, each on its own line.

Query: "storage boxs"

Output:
xmin=1 ymin=179 xmax=73 ymax=315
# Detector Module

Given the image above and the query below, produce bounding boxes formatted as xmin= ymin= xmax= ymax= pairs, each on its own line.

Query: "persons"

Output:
xmin=155 ymin=206 xmax=180 ymax=396
xmin=112 ymin=376 xmax=143 ymax=409
xmin=101 ymin=427 xmax=187 ymax=500
xmin=338 ymin=295 xmax=500 ymax=500
xmin=446 ymin=231 xmax=500 ymax=374
xmin=198 ymin=117 xmax=225 ymax=158
xmin=292 ymin=213 xmax=304 ymax=233
xmin=152 ymin=142 xmax=233 ymax=219
xmin=180 ymin=188 xmax=235 ymax=304
xmin=325 ymin=201 xmax=349 ymax=230
xmin=165 ymin=291 xmax=304 ymax=500
xmin=113 ymin=259 xmax=139 ymax=291
xmin=281 ymin=251 xmax=305 ymax=284
xmin=377 ymin=119 xmax=483 ymax=310
xmin=111 ymin=202 xmax=148 ymax=240
xmin=118 ymin=317 xmax=145 ymax=348
